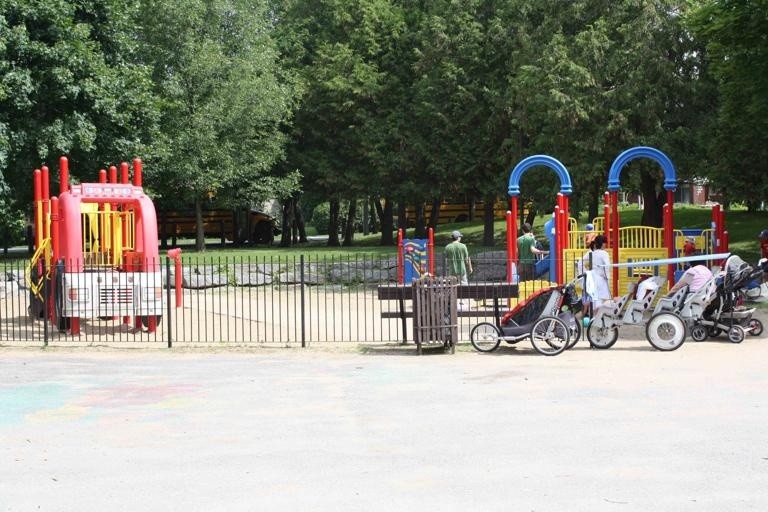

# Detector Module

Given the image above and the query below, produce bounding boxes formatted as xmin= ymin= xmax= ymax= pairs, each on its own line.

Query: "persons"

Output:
xmin=589 ymin=274 xmax=654 ymax=326
xmin=661 ymin=251 xmax=721 ymax=313
xmin=583 ymin=223 xmax=597 ymax=248
xmin=574 ymin=234 xmax=612 ymax=321
xmin=443 ymin=230 xmax=474 ymax=306
xmin=684 ymin=237 xmax=696 ymax=267
xmin=516 ymin=222 xmax=550 ymax=282
xmin=530 ymin=229 xmax=544 ymax=264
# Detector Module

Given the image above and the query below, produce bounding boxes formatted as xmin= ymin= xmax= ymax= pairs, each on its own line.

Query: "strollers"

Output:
xmin=468 ymin=272 xmax=586 ymax=356
xmin=587 ymin=253 xmax=731 ymax=351
xmin=691 ymin=254 xmax=768 ymax=344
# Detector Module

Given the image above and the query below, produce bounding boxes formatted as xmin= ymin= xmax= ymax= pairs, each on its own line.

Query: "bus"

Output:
xmin=155 ymin=191 xmax=277 ymax=245
xmin=405 ymin=178 xmax=537 ymax=228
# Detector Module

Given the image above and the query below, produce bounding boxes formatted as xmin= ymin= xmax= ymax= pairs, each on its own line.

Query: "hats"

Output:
xmin=450 ymin=231 xmax=463 ymax=238
xmin=585 ymin=225 xmax=592 ymax=230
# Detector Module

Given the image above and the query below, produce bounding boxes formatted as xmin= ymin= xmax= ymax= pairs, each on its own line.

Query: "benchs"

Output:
xmin=378 ymin=283 xmax=519 ymax=345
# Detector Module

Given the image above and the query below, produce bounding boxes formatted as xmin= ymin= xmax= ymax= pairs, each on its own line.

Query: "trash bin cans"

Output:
xmin=412 ymin=277 xmax=458 ymax=355
xmin=758 ymin=229 xmax=768 ymax=258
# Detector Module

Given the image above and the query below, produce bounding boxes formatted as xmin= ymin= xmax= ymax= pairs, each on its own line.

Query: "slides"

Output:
xmin=535 ymin=254 xmax=549 ymax=276
